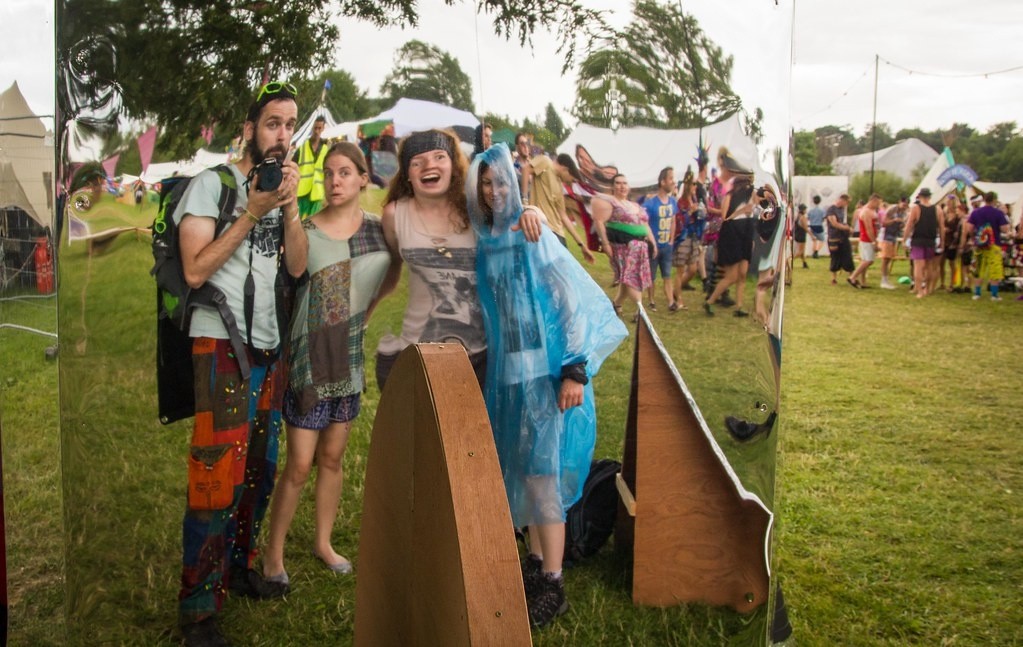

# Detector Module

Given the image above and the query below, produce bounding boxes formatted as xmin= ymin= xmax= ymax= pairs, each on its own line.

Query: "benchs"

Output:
xmin=854 ymin=254 xmax=911 ymax=281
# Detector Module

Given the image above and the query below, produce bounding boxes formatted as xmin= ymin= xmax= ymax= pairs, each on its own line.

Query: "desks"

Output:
xmin=888 ymin=237 xmax=911 ymax=276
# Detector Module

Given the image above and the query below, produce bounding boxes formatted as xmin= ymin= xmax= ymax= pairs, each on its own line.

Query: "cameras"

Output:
xmin=248 ymin=157 xmax=284 ymax=192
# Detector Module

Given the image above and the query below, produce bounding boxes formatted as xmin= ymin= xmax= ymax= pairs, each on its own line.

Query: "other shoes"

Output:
xmin=723 ymin=411 xmax=777 ymax=446
xmin=312 ymin=549 xmax=352 ymax=575
xmin=612 ymin=292 xmax=750 ymax=325
xmin=260 ymin=555 xmax=289 ymax=585
xmin=182 ymin=617 xmax=218 ymax=647
xmin=227 ymin=568 xmax=290 ymax=598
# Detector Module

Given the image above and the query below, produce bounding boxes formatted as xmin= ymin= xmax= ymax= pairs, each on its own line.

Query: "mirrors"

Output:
xmin=54 ymin=1 xmax=790 ymax=647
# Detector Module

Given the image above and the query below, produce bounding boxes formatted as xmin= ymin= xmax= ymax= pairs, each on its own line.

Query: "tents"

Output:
xmin=970 ymin=181 xmax=1022 ymax=230
xmin=0 ymin=77 xmax=53 ymax=234
xmin=833 ymin=135 xmax=940 ymax=185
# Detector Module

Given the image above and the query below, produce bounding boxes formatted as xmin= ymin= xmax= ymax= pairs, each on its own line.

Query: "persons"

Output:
xmin=846 ymin=193 xmax=885 ymax=290
xmin=112 ymin=80 xmax=784 ymax=647
xmin=807 ymin=196 xmax=826 ymax=259
xmin=849 ymin=199 xmax=892 ymax=257
xmin=904 ymin=187 xmax=1022 ymax=302
xmin=825 ymin=194 xmax=861 ymax=285
xmin=878 ymin=193 xmax=910 ymax=291
xmin=795 ymin=204 xmax=817 ymax=268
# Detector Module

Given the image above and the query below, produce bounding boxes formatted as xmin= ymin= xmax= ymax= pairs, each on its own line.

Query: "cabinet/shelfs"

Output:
xmin=614 ymin=300 xmax=774 ymax=613
xmin=352 ymin=342 xmax=534 ymax=647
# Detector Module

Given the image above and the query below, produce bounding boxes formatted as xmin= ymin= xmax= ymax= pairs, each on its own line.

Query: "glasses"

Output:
xmin=255 ymin=81 xmax=297 ymax=103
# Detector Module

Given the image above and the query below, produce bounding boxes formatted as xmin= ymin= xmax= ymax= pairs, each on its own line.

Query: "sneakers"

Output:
xmin=528 ymin=586 xmax=571 ymax=626
xmin=520 ymin=552 xmax=544 ymax=600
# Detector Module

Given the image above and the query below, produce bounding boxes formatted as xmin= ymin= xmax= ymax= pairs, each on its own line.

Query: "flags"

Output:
xmin=907 ymin=146 xmax=964 ymax=212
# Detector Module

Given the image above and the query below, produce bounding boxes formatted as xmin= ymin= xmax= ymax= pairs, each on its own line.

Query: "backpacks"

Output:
xmin=148 ymin=157 xmax=238 ymax=338
xmin=564 ymin=457 xmax=624 ymax=560
